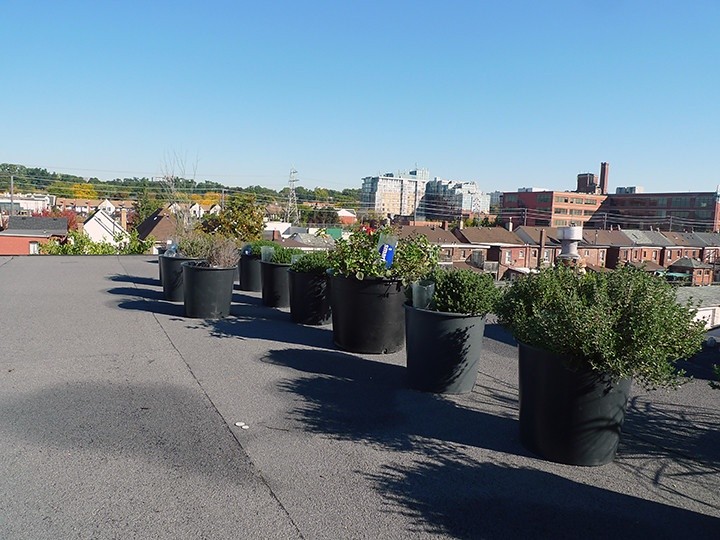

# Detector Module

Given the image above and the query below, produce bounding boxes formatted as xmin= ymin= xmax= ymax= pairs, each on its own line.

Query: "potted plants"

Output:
xmin=158 ymin=222 xmax=714 ymax=466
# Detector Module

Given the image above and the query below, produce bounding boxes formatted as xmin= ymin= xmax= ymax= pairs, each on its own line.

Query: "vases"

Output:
xmin=180 ymin=260 xmax=237 ymax=319
xmin=157 ymin=246 xmax=169 ymax=281
